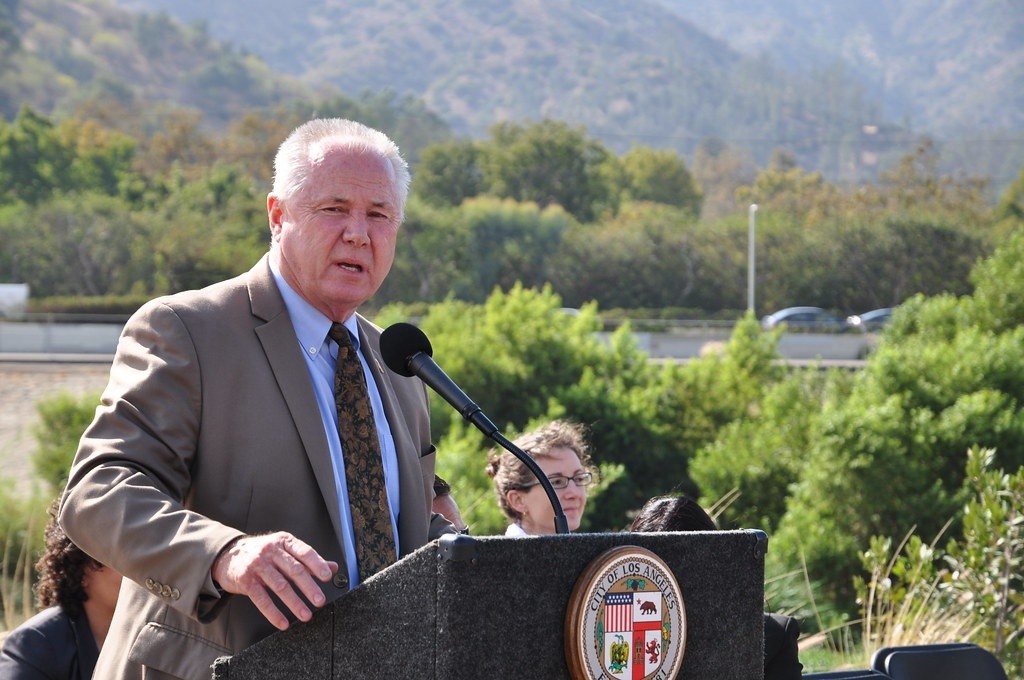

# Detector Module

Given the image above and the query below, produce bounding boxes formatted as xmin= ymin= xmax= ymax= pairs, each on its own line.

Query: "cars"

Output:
xmin=850 ymin=307 xmax=898 ymax=330
xmin=758 ymin=305 xmax=872 ymax=360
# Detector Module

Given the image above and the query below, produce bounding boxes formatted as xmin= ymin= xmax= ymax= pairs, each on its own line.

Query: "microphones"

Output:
xmin=379 ymin=323 xmax=497 ymax=438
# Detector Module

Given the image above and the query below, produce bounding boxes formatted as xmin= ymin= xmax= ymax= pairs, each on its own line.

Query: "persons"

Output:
xmin=0 ymin=489 xmax=123 ymax=680
xmin=58 ymin=119 xmax=437 ymax=680
xmin=631 ymin=495 xmax=803 ymax=680
xmin=430 ymin=475 xmax=470 ymax=535
xmin=485 ymin=422 xmax=594 ymax=538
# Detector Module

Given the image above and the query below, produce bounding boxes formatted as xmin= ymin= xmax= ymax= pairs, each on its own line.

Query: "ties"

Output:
xmin=326 ymin=320 xmax=397 ymax=585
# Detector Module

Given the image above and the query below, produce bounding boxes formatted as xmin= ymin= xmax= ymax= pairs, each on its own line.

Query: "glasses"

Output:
xmin=458 ymin=525 xmax=471 ymax=535
xmin=518 ymin=472 xmax=593 ymax=490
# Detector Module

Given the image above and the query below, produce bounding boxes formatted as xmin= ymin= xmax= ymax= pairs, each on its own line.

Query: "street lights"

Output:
xmin=747 ymin=202 xmax=760 ymax=315
